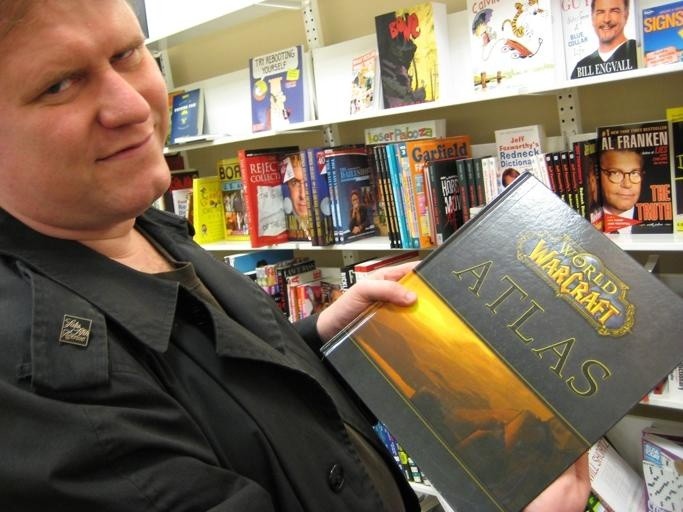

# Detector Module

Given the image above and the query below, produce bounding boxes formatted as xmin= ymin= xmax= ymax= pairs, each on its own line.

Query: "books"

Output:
xmin=156 ymin=109 xmax=683 ymax=250
xmin=348 ymin=1 xmax=439 ymax=114
xmin=588 ymin=254 xmax=683 ymax=512
xmin=165 ymin=87 xmax=227 ymax=145
xmin=249 ymin=45 xmax=307 ymax=132
xmin=466 ymin=0 xmax=683 ymax=95
xmin=374 ymin=423 xmax=443 ymax=512
xmin=320 ymin=171 xmax=683 ymax=512
xmin=225 ymin=250 xmax=420 ymax=323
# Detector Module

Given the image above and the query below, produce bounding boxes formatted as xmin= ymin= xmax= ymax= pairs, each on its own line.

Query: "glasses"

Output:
xmin=598 ymin=165 xmax=647 ymax=185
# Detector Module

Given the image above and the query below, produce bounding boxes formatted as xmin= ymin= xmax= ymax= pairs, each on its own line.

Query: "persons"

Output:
xmin=1 ymin=0 xmax=592 ymax=512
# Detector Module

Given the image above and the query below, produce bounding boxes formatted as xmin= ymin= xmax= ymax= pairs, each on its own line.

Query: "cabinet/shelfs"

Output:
xmin=142 ymin=0 xmax=683 ymax=511
